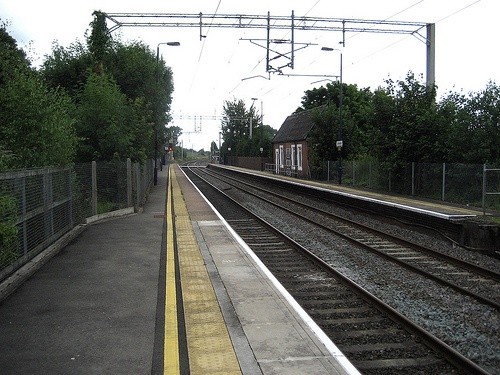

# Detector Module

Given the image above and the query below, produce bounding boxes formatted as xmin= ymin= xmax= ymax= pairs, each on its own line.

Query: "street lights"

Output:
xmin=250 ymin=97 xmax=264 ymax=171
xmin=321 ymin=47 xmax=343 ymax=186
xmin=153 ymin=42 xmax=181 ymax=186
